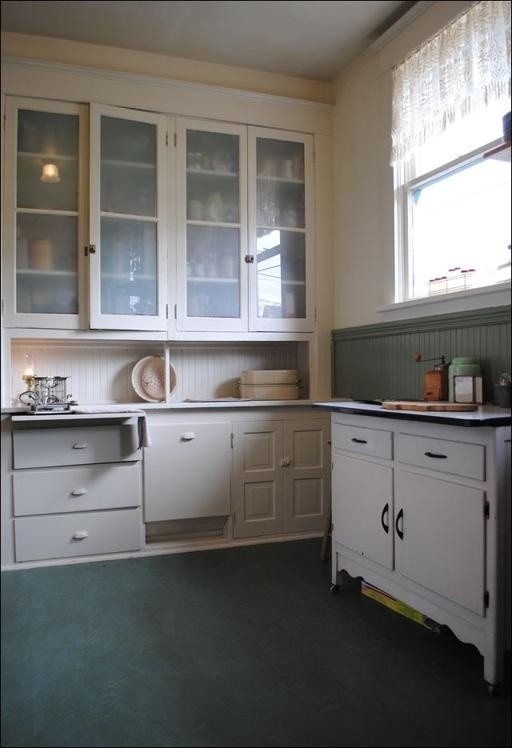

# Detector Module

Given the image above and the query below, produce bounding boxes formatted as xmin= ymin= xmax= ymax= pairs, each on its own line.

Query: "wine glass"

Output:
xmin=22 ymin=351 xmax=36 ymax=392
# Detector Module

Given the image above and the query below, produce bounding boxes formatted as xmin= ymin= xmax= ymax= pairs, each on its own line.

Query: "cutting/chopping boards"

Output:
xmin=382 ymin=399 xmax=479 ymax=411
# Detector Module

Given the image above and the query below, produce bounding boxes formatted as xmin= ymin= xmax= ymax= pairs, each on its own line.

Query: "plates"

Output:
xmin=131 ymin=354 xmax=176 ymax=403
